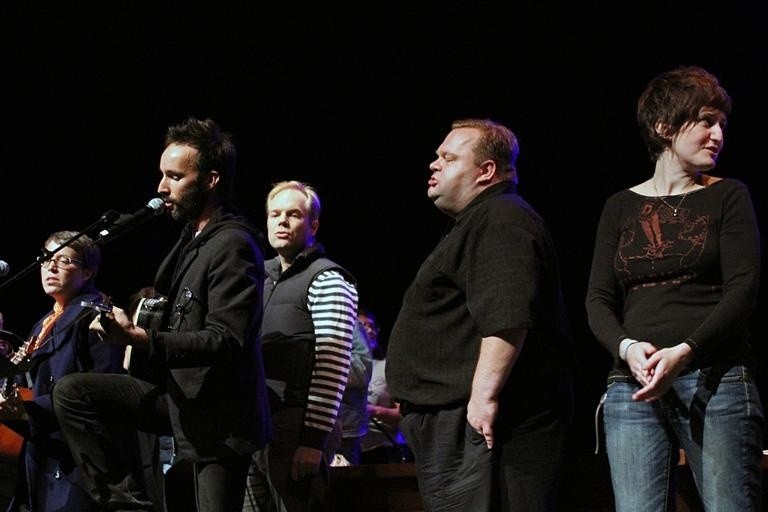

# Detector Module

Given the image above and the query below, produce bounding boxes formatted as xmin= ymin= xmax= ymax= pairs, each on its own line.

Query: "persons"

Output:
xmin=242 ymin=181 xmax=358 ymax=512
xmin=385 ymin=119 xmax=566 ymax=512
xmin=339 ymin=310 xmax=404 ymax=466
xmin=586 ymin=66 xmax=768 ymax=512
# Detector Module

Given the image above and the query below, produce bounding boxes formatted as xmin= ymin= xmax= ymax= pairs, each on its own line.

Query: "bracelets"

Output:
xmin=620 ymin=340 xmax=638 ymax=360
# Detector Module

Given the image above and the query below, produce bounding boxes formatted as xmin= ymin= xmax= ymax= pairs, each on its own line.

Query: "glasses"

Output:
xmin=40 ymin=255 xmax=83 ymax=267
xmin=168 ymin=285 xmax=193 ymax=329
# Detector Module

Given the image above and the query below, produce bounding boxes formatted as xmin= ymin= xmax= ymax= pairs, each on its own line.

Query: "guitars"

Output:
xmin=0 ymin=335 xmax=40 ymax=459
xmin=82 ymin=296 xmax=168 ymax=377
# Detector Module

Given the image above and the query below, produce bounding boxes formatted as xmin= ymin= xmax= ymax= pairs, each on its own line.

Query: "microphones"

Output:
xmin=89 ymin=196 xmax=166 ymax=246
xmin=0 ymin=260 xmax=10 ymax=278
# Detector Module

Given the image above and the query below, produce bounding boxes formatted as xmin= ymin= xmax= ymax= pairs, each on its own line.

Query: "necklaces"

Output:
xmin=654 ymin=175 xmax=696 ymax=217
